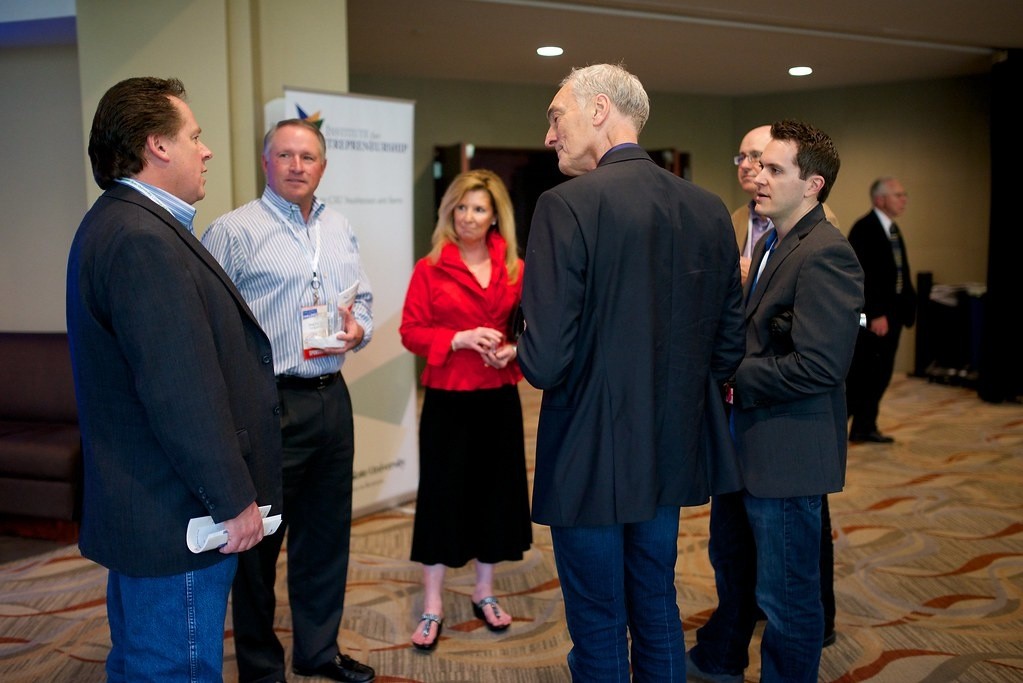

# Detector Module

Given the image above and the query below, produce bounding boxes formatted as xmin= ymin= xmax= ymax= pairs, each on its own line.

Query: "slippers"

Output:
xmin=471 ymin=597 xmax=510 ymax=632
xmin=413 ymin=614 xmax=443 ymax=651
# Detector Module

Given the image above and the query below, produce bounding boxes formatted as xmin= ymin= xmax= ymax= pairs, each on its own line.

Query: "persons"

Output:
xmin=846 ymin=173 xmax=916 ymax=445
xmin=517 ymin=64 xmax=747 ymax=683
xmin=398 ymin=169 xmax=525 ymax=653
xmin=66 ymin=75 xmax=377 ymax=683
xmin=686 ymin=120 xmax=865 ymax=683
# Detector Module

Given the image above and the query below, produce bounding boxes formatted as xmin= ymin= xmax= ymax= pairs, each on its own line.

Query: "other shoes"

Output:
xmin=849 ymin=431 xmax=895 ymax=444
xmin=822 ymin=630 xmax=837 ymax=648
xmin=682 ymin=652 xmax=745 ymax=683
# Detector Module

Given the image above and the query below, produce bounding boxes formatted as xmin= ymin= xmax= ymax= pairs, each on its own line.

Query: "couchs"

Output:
xmin=0 ymin=329 xmax=90 ymax=544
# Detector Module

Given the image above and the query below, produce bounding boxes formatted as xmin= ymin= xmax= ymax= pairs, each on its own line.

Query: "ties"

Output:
xmin=890 ymin=224 xmax=904 ymax=293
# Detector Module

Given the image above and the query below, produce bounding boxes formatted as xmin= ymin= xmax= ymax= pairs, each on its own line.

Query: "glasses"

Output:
xmin=733 ymin=152 xmax=762 ymax=166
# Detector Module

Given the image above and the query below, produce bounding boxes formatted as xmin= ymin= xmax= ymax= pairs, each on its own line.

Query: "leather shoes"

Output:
xmin=293 ymin=650 xmax=375 ymax=683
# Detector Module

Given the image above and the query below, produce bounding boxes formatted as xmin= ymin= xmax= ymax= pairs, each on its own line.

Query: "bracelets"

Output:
xmin=512 ymin=345 xmax=517 ymax=361
